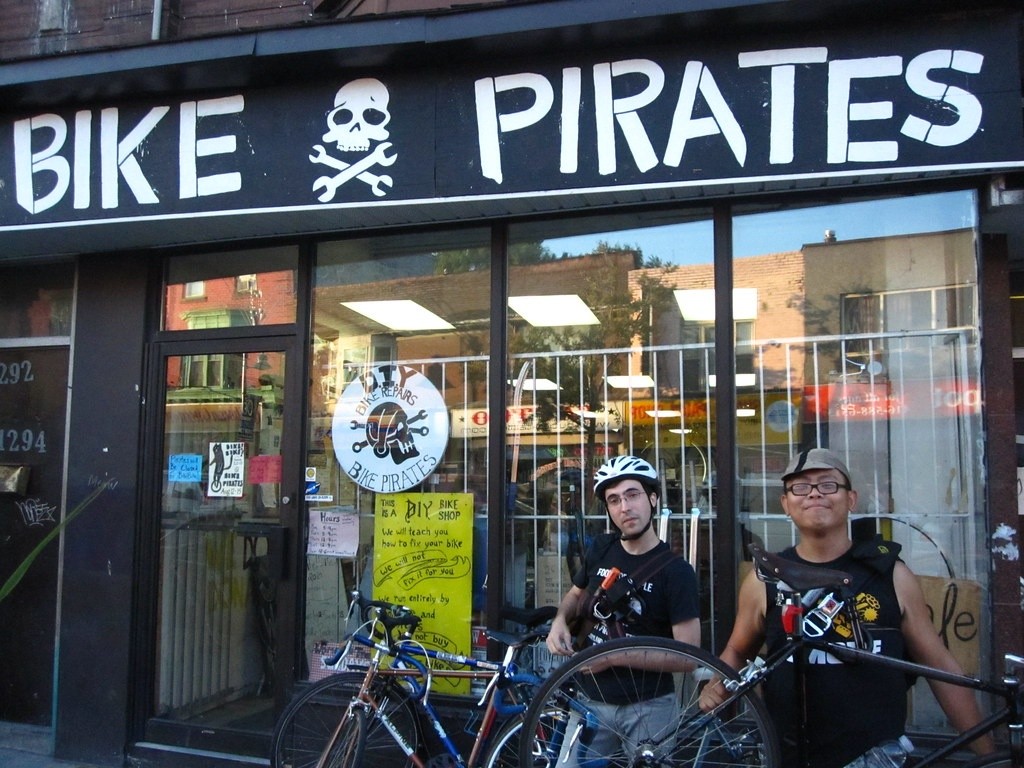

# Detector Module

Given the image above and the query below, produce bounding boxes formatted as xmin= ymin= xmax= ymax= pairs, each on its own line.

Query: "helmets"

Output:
xmin=594 ymin=456 xmax=660 ymax=498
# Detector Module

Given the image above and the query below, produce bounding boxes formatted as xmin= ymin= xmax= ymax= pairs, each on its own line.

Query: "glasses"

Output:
xmin=608 ymin=490 xmax=646 ymax=503
xmin=786 ymin=481 xmax=848 ymax=496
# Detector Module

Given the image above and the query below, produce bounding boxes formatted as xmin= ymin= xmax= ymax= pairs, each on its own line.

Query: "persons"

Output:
xmin=544 ymin=453 xmax=706 ymax=768
xmin=693 ymin=447 xmax=994 ymax=768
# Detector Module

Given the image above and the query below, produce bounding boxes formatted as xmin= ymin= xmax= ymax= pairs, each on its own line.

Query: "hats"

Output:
xmin=781 ymin=449 xmax=852 ymax=488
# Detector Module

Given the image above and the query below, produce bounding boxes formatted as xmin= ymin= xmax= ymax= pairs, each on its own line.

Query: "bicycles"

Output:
xmin=269 ymin=589 xmax=559 ymax=768
xmin=517 ymin=542 xmax=1022 ymax=767
xmin=313 ymin=630 xmax=564 ymax=768
